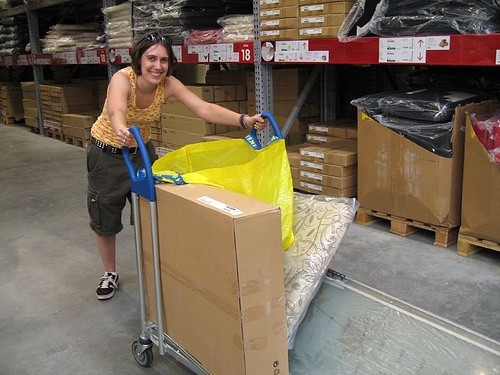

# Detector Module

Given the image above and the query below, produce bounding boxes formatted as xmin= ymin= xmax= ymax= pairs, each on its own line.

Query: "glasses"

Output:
xmin=135 ymin=33 xmax=173 ymax=48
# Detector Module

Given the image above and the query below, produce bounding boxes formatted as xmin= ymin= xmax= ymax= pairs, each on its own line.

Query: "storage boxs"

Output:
xmin=139 ymin=182 xmax=289 ymax=375
xmin=0 ymin=1 xmax=500 ymax=243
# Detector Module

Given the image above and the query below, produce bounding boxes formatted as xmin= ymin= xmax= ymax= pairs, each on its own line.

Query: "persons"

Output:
xmin=87 ymin=28 xmax=268 ymax=300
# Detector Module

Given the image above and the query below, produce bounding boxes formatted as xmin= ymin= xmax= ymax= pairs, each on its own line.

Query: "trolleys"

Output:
xmin=122 ymin=111 xmax=285 ymax=375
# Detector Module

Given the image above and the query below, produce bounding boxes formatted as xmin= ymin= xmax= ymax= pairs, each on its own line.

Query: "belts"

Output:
xmin=91 ymin=135 xmax=152 ymax=155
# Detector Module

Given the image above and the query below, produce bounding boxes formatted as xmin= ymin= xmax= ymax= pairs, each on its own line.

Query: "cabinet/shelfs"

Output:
xmin=0 ymin=1 xmax=117 ymax=135
xmin=105 ymin=0 xmax=328 ymax=172
xmin=256 ymin=1 xmax=500 ymax=152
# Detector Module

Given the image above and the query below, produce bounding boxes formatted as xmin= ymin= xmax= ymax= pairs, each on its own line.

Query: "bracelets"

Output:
xmin=239 ymin=114 xmax=250 ymax=129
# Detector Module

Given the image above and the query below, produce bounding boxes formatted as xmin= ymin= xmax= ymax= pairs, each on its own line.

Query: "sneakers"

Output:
xmin=95 ymin=272 xmax=120 ymax=300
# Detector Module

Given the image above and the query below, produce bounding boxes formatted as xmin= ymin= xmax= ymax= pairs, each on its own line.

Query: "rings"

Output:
xmin=123 ymin=140 xmax=126 ymax=143
xmin=125 ymin=136 xmax=127 ymax=139
xmin=122 ymin=130 xmax=127 ymax=135
xmin=123 ymin=143 xmax=125 ymax=145
